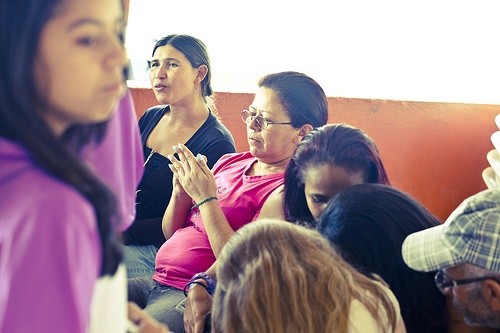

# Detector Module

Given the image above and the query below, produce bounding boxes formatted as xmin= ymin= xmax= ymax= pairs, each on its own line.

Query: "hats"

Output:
xmin=402 ymin=188 xmax=500 ymax=273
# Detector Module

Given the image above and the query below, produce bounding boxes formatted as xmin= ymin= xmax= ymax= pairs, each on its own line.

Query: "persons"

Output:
xmin=118 ymin=32 xmax=236 ymax=278
xmin=401 ymin=189 xmax=500 ymax=329
xmin=182 ymin=123 xmax=443 ymax=333
xmin=124 ymin=71 xmax=327 ymax=333
xmin=0 ymin=0 xmax=173 ymax=333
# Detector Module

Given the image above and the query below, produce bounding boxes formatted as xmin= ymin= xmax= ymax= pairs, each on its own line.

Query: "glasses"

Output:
xmin=434 ymin=271 xmax=500 ymax=296
xmin=240 ymin=110 xmax=292 ymax=129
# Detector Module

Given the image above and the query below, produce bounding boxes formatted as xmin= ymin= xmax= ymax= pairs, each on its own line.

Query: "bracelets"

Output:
xmin=190 ymin=272 xmax=216 ymax=293
xmin=182 ymin=281 xmax=210 ymax=297
xmin=190 ymin=196 xmax=217 ymax=214
